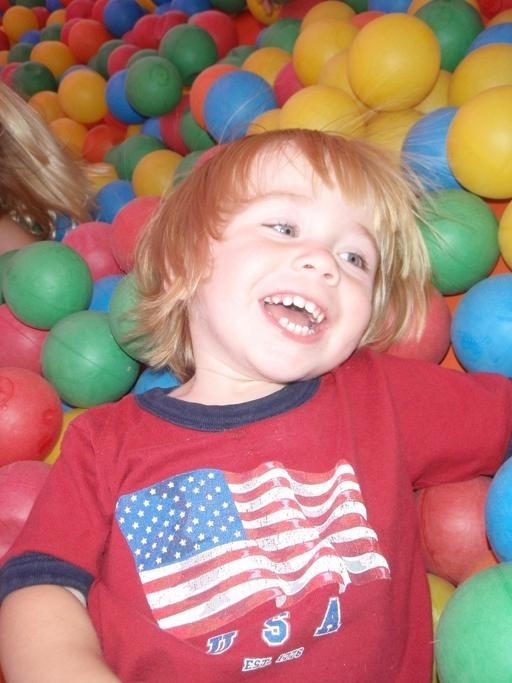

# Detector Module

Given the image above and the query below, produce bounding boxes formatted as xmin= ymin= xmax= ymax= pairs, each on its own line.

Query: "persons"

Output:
xmin=1 ymin=127 xmax=512 ymax=683
xmin=0 ymin=83 xmax=100 ymax=255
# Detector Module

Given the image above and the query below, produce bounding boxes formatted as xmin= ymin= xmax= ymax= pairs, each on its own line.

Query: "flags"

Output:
xmin=115 ymin=457 xmax=391 ymax=640
